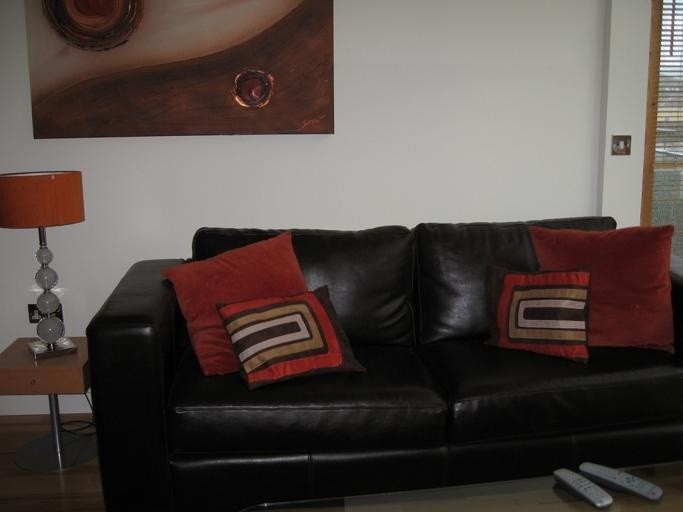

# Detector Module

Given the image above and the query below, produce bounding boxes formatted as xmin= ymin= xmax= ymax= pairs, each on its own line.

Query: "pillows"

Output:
xmin=190 ymin=226 xmax=420 ymax=361
xmin=161 ymin=230 xmax=309 ymax=376
xmin=217 ymin=284 xmax=367 ymax=390
xmin=528 ymin=223 xmax=674 ymax=355
xmin=414 ymin=216 xmax=618 ymax=349
xmin=485 ymin=260 xmax=594 ymax=367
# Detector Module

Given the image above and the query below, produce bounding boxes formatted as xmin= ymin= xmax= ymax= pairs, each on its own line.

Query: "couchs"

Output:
xmin=85 ymin=216 xmax=683 ymax=512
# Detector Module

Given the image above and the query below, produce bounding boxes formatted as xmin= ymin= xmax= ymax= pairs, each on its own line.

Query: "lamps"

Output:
xmin=0 ymin=170 xmax=85 ymax=358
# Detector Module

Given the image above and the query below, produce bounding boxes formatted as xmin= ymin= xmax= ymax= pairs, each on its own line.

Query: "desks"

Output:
xmin=0 ymin=337 xmax=98 ymax=472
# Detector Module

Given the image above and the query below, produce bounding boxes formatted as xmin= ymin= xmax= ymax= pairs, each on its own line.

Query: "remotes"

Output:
xmin=552 ymin=466 xmax=616 ymax=510
xmin=573 ymin=461 xmax=663 ymax=503
